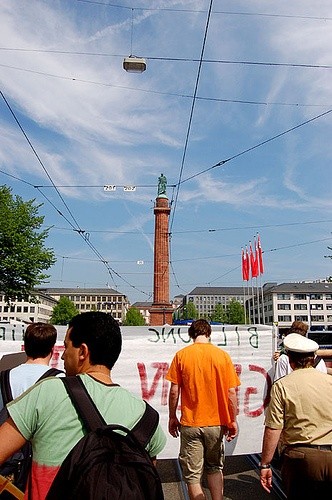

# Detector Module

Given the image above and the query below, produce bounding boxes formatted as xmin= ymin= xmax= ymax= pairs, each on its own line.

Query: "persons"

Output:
xmin=273 ymin=321 xmax=327 ymax=457
xmin=0 ymin=322 xmax=67 ymax=461
xmin=165 ymin=319 xmax=241 ymax=500
xmin=0 ymin=311 xmax=167 ymax=500
xmin=259 ymin=334 xmax=332 ymax=500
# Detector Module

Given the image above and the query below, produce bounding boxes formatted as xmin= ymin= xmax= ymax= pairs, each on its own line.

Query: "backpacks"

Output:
xmin=0 ymin=368 xmax=64 ymax=491
xmin=28 ymin=375 xmax=165 ymax=500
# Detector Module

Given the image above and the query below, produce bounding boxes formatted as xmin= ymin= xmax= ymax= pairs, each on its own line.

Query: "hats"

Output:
xmin=283 ymin=333 xmax=319 ymax=356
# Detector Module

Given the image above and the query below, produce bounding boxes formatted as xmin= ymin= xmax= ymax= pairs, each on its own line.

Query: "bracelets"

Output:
xmin=260 ymin=464 xmax=271 ymax=468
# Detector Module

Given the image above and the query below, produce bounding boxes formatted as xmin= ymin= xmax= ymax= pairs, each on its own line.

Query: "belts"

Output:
xmin=289 ymin=443 xmax=332 ymax=450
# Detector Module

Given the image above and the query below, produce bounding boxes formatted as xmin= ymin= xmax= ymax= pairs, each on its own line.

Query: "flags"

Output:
xmin=241 ymin=232 xmax=263 ymax=281
